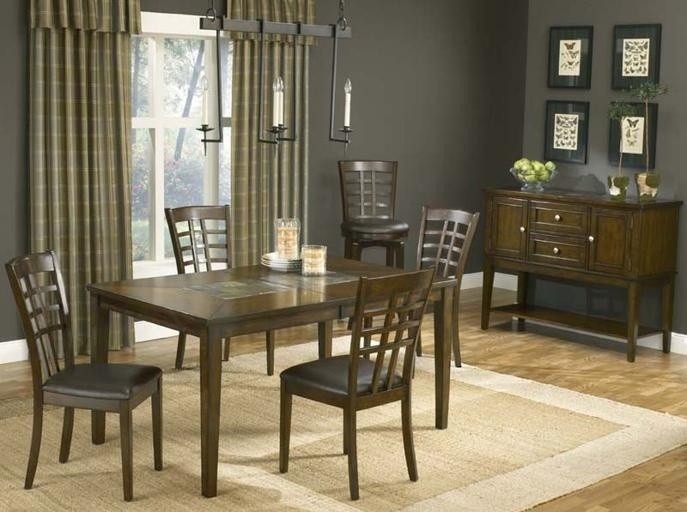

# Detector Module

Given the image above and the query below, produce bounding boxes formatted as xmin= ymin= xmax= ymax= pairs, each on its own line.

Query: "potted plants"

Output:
xmin=620 ymin=76 xmax=673 ymax=203
xmin=604 ymin=99 xmax=636 ymax=202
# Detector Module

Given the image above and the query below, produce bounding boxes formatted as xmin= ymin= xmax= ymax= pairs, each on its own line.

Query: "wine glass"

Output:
xmin=509 ymin=168 xmax=559 ymax=191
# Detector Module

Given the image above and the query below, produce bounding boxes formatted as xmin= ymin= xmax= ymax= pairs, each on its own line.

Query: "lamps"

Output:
xmin=187 ymin=0 xmax=358 ymax=162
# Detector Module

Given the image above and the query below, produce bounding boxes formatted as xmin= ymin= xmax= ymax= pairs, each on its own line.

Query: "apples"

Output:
xmin=513 ymin=157 xmax=556 ymax=184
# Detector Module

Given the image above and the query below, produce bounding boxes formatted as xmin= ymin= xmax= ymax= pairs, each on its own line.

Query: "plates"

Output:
xmin=260 ymin=252 xmax=301 ymax=274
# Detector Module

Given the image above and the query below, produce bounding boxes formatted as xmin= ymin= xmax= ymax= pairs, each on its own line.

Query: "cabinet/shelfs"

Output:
xmin=476 ymin=184 xmax=682 ymax=368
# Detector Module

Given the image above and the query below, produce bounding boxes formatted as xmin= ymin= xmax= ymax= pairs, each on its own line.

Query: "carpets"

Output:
xmin=2 ymin=324 xmax=684 ymax=512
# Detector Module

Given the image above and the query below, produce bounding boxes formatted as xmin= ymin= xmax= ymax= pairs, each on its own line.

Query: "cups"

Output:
xmin=301 ymin=244 xmax=326 ymax=276
xmin=274 ymin=217 xmax=299 ymax=258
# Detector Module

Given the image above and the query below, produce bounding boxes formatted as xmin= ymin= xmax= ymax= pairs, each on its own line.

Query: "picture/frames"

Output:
xmin=610 ymin=24 xmax=663 ymax=93
xmin=543 ymin=25 xmax=594 ymax=92
xmin=606 ymin=100 xmax=658 ymax=170
xmin=540 ymin=96 xmax=590 ymax=167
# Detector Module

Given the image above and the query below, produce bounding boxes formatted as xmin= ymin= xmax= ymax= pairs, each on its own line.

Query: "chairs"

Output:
xmin=2 ymin=245 xmax=166 ymax=501
xmin=272 ymin=258 xmax=433 ymax=503
xmin=332 ymin=157 xmax=410 ymax=332
xmin=380 ymin=201 xmax=484 ymax=370
xmin=157 ymin=199 xmax=279 ymax=378
